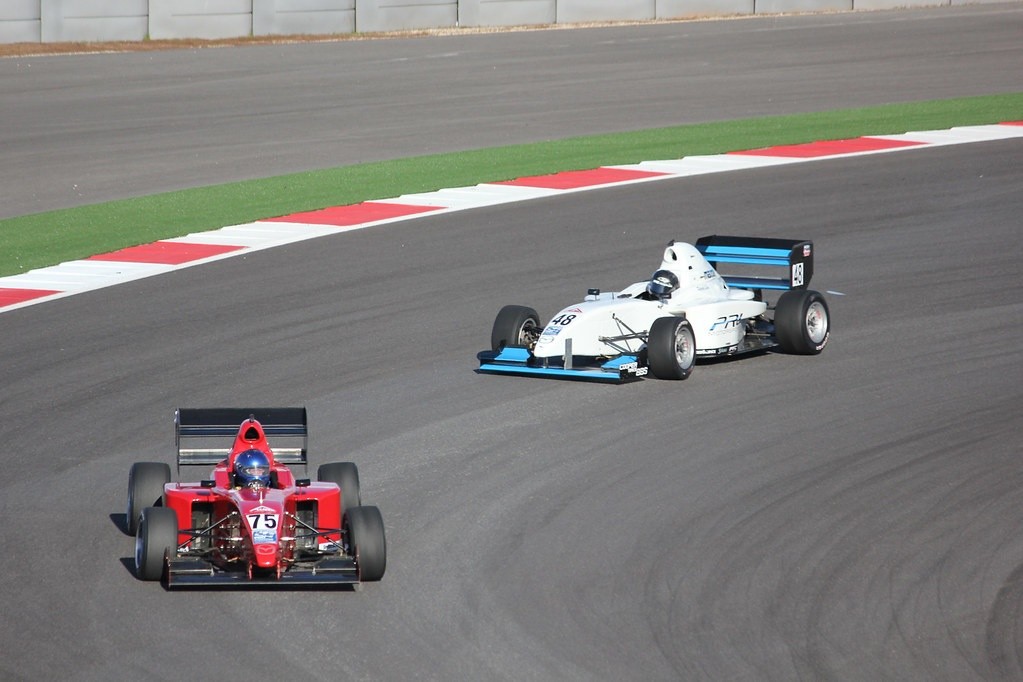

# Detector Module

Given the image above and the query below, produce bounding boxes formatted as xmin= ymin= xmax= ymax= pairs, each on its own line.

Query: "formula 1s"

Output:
xmin=121 ymin=407 xmax=386 ymax=589
xmin=476 ymin=233 xmax=832 ymax=385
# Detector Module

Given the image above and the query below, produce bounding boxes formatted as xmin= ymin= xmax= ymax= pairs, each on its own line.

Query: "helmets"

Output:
xmin=647 ymin=271 xmax=680 ymax=300
xmin=233 ymin=449 xmax=270 ymax=487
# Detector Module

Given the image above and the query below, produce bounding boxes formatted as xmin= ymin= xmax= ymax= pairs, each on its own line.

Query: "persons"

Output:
xmin=229 ymin=450 xmax=274 ymax=490
xmin=646 ymin=269 xmax=679 ymax=300
